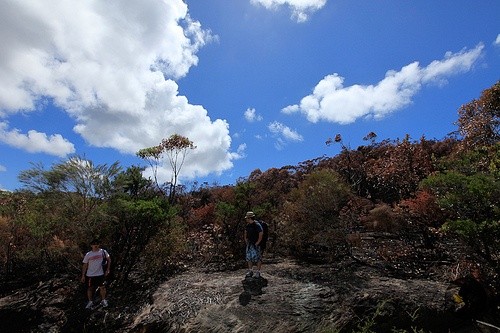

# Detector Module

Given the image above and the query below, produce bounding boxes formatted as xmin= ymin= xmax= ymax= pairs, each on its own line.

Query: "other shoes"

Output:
xmin=246 ymin=270 xmax=254 ymax=276
xmin=255 ymin=271 xmax=260 ymax=278
xmin=100 ymin=298 xmax=109 ymax=308
xmin=86 ymin=300 xmax=95 ymax=309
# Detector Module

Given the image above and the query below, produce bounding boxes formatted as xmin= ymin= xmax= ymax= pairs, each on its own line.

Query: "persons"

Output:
xmin=243 ymin=211 xmax=264 ymax=278
xmin=80 ymin=239 xmax=111 ymax=310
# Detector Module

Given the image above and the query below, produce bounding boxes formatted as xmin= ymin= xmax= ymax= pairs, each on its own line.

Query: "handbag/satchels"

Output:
xmin=101 ymin=249 xmax=108 ymax=275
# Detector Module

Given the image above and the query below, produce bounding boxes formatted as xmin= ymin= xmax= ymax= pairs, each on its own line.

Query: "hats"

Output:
xmin=245 ymin=211 xmax=255 ymax=218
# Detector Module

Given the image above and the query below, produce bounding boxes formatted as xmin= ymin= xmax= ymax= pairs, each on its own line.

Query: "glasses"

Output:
xmin=92 ymin=242 xmax=99 ymax=245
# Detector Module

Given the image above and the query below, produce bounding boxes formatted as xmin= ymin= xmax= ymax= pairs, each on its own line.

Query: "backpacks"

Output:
xmin=258 ymin=220 xmax=270 ymax=242
